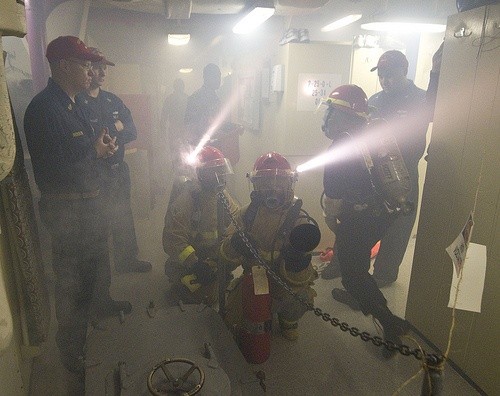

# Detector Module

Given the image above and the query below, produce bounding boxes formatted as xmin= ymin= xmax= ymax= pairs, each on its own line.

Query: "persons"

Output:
xmin=320 ymin=84 xmax=411 ymax=358
xmin=75 ymin=47 xmax=153 ymax=272
xmin=221 ymin=152 xmax=317 ymax=362
xmin=23 ymin=35 xmax=132 ymax=373
xmin=367 ymin=50 xmax=428 ymax=286
xmin=185 ymin=63 xmax=244 ymax=142
xmin=162 ymin=147 xmax=243 ymax=306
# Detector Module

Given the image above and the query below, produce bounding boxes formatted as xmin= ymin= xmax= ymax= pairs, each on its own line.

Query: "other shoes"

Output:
xmin=55 ymin=331 xmax=85 ymax=372
xmin=371 ymin=274 xmax=394 ymax=288
xmin=382 ymin=316 xmax=411 ymax=360
xmin=321 ymin=259 xmax=341 ymax=279
xmin=332 ymin=287 xmax=362 ymax=312
xmin=88 ymin=297 xmax=132 ymax=321
xmin=283 ymin=327 xmax=298 ymax=340
xmin=115 ymin=257 xmax=152 ymax=274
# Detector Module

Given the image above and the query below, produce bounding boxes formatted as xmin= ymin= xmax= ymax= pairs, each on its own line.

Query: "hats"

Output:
xmin=370 ymin=49 xmax=409 ymax=72
xmin=86 ymin=46 xmax=116 ymax=67
xmin=45 ymin=36 xmax=105 ymax=63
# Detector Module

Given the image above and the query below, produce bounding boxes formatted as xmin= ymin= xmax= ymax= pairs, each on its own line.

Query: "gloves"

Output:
xmin=279 ymin=245 xmax=312 ymax=272
xmin=188 ymin=258 xmax=211 ymax=288
xmin=322 ymin=196 xmax=344 ymax=235
xmin=230 ymin=232 xmax=258 ymax=259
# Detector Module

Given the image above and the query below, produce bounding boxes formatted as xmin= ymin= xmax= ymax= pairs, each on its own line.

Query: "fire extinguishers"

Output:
xmin=240 ymin=256 xmax=272 ymax=364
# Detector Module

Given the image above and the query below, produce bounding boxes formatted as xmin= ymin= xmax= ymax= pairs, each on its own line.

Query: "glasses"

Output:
xmin=64 ymin=57 xmax=93 ymax=72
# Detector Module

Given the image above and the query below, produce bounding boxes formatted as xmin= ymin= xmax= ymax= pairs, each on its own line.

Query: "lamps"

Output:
xmin=233 ymin=1 xmax=276 ymax=34
xmin=166 ymin=30 xmax=191 ymax=47
xmin=320 ymin=11 xmax=362 ymax=34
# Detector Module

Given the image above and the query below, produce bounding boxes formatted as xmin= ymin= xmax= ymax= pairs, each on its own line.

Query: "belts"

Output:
xmin=40 ymin=188 xmax=102 ymax=201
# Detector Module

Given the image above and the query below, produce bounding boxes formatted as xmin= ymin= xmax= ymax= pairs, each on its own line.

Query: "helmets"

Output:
xmin=250 ymin=152 xmax=293 ymax=178
xmin=326 ymin=84 xmax=369 ymax=121
xmin=196 ymin=145 xmax=226 ymax=168
